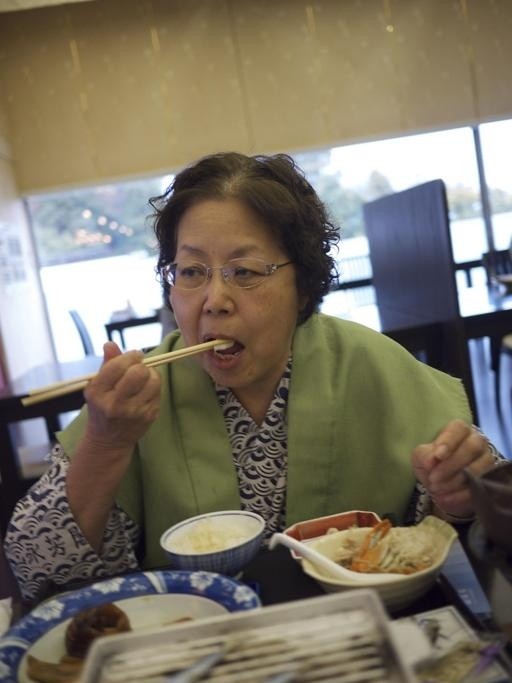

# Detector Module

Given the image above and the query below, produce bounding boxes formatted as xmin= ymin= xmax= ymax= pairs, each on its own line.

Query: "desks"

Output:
xmin=104 ymin=312 xmax=161 ymax=353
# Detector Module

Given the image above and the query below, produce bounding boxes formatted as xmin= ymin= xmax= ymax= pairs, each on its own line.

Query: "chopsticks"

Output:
xmin=22 ymin=336 xmax=233 ymax=409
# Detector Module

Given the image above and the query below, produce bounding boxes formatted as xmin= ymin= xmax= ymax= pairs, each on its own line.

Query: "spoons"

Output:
xmin=269 ymin=532 xmax=406 ymax=580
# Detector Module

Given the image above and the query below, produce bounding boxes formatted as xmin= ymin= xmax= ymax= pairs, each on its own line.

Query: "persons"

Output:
xmin=3 ymin=152 xmax=503 ymax=604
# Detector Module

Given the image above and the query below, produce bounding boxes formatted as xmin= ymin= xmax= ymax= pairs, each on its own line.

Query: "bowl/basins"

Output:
xmin=301 ymin=515 xmax=459 ymax=612
xmin=160 ymin=510 xmax=265 ymax=581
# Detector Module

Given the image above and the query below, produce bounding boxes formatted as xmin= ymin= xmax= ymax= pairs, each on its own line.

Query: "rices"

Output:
xmin=167 ymin=514 xmax=259 ymax=554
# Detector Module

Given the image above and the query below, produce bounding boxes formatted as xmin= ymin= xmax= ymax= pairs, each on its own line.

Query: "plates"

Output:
xmin=0 ymin=570 xmax=262 ymax=683
xmin=281 ymin=510 xmax=385 ymax=561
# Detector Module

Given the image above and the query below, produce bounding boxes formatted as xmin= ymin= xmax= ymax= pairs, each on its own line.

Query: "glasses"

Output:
xmin=157 ymin=255 xmax=299 ymax=290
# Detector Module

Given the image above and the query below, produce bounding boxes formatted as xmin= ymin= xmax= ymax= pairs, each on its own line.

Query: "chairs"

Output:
xmin=483 ymin=252 xmax=512 ymax=372
xmin=69 ymin=308 xmax=94 ymax=355
